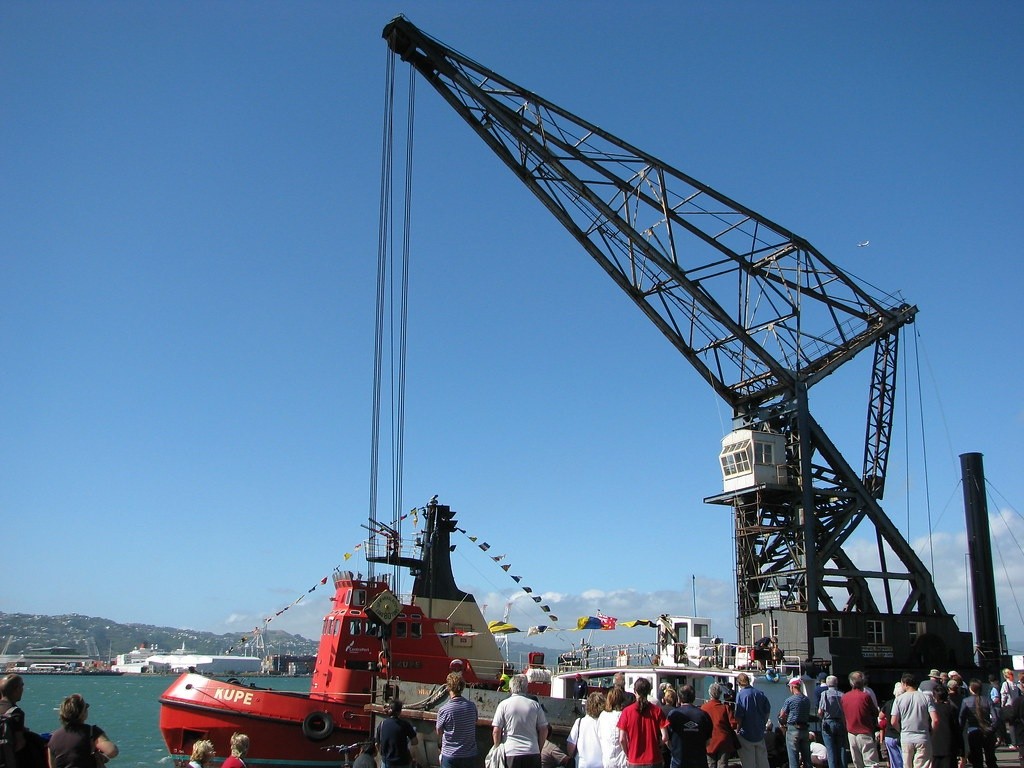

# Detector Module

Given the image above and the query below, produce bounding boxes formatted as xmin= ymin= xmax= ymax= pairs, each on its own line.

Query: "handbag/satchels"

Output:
xmin=90 ymin=726 xmax=109 ymax=768
xmin=1000 ymin=705 xmax=1012 ymax=722
xmin=566 ymin=758 xmax=575 ymax=768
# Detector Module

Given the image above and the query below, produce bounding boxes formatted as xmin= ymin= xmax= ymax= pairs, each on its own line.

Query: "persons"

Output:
xmin=186 ymin=740 xmax=216 ymax=768
xmin=483 ymin=673 xmax=570 ymax=768
xmin=352 ymin=743 xmax=378 ymax=768
xmin=435 ymin=672 xmax=478 ymax=768
xmin=564 ymin=673 xmax=670 ymax=768
xmin=777 ymin=669 xmax=1024 ymax=768
xmin=0 ymin=674 xmax=50 ymax=768
xmin=385 ymin=676 xmax=400 ymax=704
xmin=47 ymin=693 xmax=118 ymax=768
xmin=376 ymin=700 xmax=418 ymax=768
xmin=754 ymin=636 xmax=778 ymax=662
xmin=659 ymin=674 xmax=776 ymax=768
xmin=220 ymin=732 xmax=250 ymax=768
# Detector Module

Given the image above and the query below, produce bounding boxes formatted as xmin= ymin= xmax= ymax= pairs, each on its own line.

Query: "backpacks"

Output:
xmin=0 ymin=706 xmax=19 ymax=768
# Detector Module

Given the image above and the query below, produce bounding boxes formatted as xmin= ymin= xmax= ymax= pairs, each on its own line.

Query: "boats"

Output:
xmin=159 ymin=495 xmax=649 ymax=768
xmin=553 ymin=574 xmax=804 ymax=705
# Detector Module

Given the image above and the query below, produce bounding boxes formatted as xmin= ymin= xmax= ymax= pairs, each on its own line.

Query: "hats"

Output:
xmin=927 ymin=669 xmax=942 ymax=678
xmin=575 ymin=674 xmax=581 ymax=678
xmin=787 ymin=678 xmax=801 ymax=687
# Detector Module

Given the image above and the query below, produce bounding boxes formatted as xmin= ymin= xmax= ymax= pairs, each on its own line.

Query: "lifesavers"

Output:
xmin=765 ymin=668 xmax=781 ymax=684
xmin=302 ymin=710 xmax=334 ymax=742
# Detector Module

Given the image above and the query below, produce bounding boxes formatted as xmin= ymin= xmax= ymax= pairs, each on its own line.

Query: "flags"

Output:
xmin=344 ymin=506 xmax=423 ymax=560
xmin=455 ymin=527 xmax=559 ymax=622
xmin=577 ymin=615 xmax=659 ymax=631
xmin=242 ymin=577 xmax=329 ymax=644
xmin=439 ymin=621 xmax=552 ymax=638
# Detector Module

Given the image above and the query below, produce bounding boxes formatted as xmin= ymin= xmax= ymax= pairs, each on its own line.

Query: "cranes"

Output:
xmin=367 ymin=14 xmax=978 ymax=681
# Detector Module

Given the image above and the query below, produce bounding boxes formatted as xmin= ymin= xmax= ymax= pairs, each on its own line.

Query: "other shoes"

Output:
xmin=1009 ymin=746 xmax=1019 ymax=751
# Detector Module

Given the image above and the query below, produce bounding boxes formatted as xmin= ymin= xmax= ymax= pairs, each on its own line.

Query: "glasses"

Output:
xmin=84 ymin=703 xmax=89 ymax=710
